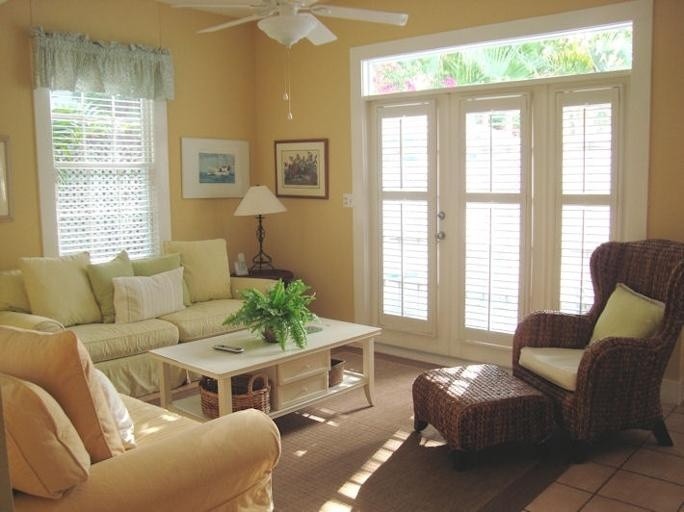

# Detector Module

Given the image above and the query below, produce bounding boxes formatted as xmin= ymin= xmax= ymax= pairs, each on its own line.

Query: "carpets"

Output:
xmin=147 ymin=345 xmax=595 ymax=512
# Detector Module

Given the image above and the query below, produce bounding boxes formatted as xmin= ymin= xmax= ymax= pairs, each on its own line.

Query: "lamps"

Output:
xmin=233 ymin=185 xmax=287 ymax=277
xmin=257 ymin=14 xmax=316 ymax=121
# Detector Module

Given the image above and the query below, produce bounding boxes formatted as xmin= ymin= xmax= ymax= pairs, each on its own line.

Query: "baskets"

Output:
xmin=199 ymin=373 xmax=273 ymax=420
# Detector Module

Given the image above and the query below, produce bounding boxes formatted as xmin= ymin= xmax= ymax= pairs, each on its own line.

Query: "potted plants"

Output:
xmin=221 ymin=277 xmax=319 ymax=348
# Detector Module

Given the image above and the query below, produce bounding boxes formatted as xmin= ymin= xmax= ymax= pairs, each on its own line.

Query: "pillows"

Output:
xmin=18 ymin=250 xmax=104 ymax=326
xmin=586 ymin=282 xmax=666 ymax=353
xmin=131 ymin=255 xmax=191 ymax=307
xmin=1 ymin=374 xmax=89 ymax=500
xmin=96 ymin=369 xmax=136 ymax=451
xmin=113 ymin=267 xmax=184 ymax=322
xmin=158 ymin=240 xmax=232 ymax=302
xmin=0 ymin=325 xmax=136 ymax=463
xmin=87 ymin=250 xmax=133 ymax=322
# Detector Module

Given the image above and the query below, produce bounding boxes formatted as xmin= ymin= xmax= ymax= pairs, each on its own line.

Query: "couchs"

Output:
xmin=1 ymin=254 xmax=286 ymax=398
xmin=0 ymin=324 xmax=283 ymax=512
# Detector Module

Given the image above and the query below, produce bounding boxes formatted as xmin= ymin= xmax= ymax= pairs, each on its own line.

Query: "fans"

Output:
xmin=171 ymin=0 xmax=408 ymax=46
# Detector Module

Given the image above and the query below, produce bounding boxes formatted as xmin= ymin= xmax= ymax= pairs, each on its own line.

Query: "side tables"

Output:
xmin=231 ymin=270 xmax=293 ymax=288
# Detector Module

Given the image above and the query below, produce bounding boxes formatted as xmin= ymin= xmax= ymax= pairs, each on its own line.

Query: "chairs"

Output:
xmin=513 ymin=241 xmax=682 ymax=461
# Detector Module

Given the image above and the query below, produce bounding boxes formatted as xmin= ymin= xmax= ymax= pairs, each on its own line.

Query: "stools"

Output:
xmin=412 ymin=365 xmax=556 ymax=467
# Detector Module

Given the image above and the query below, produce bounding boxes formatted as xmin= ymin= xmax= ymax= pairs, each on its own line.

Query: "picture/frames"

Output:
xmin=0 ymin=136 xmax=16 ymax=224
xmin=274 ymin=139 xmax=328 ymax=199
xmin=181 ymin=137 xmax=249 ymax=199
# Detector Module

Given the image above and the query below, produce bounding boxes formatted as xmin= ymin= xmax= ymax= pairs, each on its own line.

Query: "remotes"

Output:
xmin=212 ymin=344 xmax=245 ymax=353
